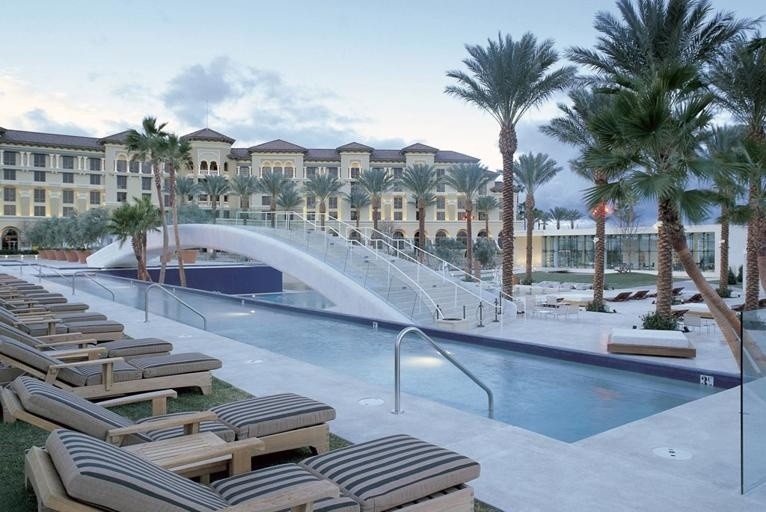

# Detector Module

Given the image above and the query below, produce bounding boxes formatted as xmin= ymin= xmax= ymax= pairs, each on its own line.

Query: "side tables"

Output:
xmin=119 ymin=431 xmax=233 ymax=484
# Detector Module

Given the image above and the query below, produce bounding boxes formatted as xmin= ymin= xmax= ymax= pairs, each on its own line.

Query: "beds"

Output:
xmin=607 ymin=327 xmax=697 ymax=358
xmin=672 ymin=302 xmax=731 ymax=318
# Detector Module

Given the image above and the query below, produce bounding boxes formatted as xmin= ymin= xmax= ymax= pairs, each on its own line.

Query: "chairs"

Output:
xmin=732 ymin=299 xmax=766 ymax=312
xmin=523 ymin=296 xmax=581 ymax=321
xmin=24 ymin=428 xmax=481 ymax=512
xmin=0 ymin=273 xmax=221 ymax=397
xmin=513 ymin=281 xmax=593 ymax=295
xmin=1 ymin=375 xmax=337 ymax=459
xmin=608 ymin=287 xmax=705 ymax=303
xmin=642 ymin=262 xmax=655 ymax=270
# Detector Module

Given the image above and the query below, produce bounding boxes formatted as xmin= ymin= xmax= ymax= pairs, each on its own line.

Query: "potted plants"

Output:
xmin=24 ymin=208 xmax=112 ymax=264
xmin=167 ymin=203 xmax=213 ymax=264
